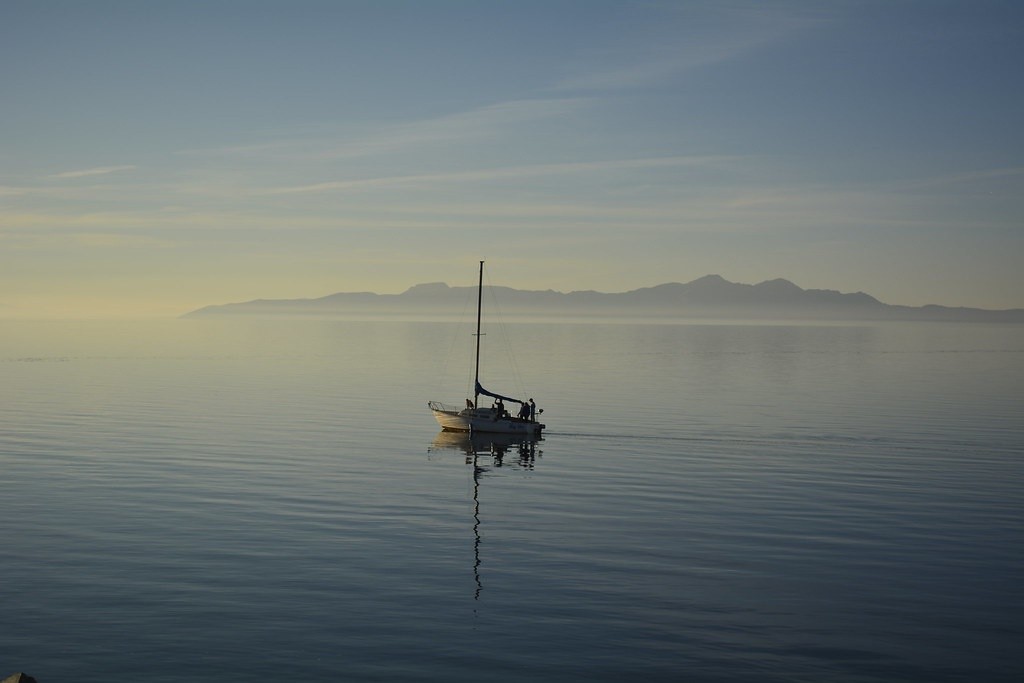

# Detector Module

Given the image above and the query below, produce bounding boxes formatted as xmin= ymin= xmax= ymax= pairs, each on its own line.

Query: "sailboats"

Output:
xmin=427 ymin=428 xmax=546 ymax=600
xmin=428 ymin=261 xmax=546 ymax=434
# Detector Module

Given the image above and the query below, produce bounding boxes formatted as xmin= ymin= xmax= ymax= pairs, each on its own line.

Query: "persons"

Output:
xmin=466 ymin=399 xmax=474 ymax=409
xmin=517 ymin=398 xmax=535 ymax=422
xmin=494 ymin=398 xmax=504 ymax=417
